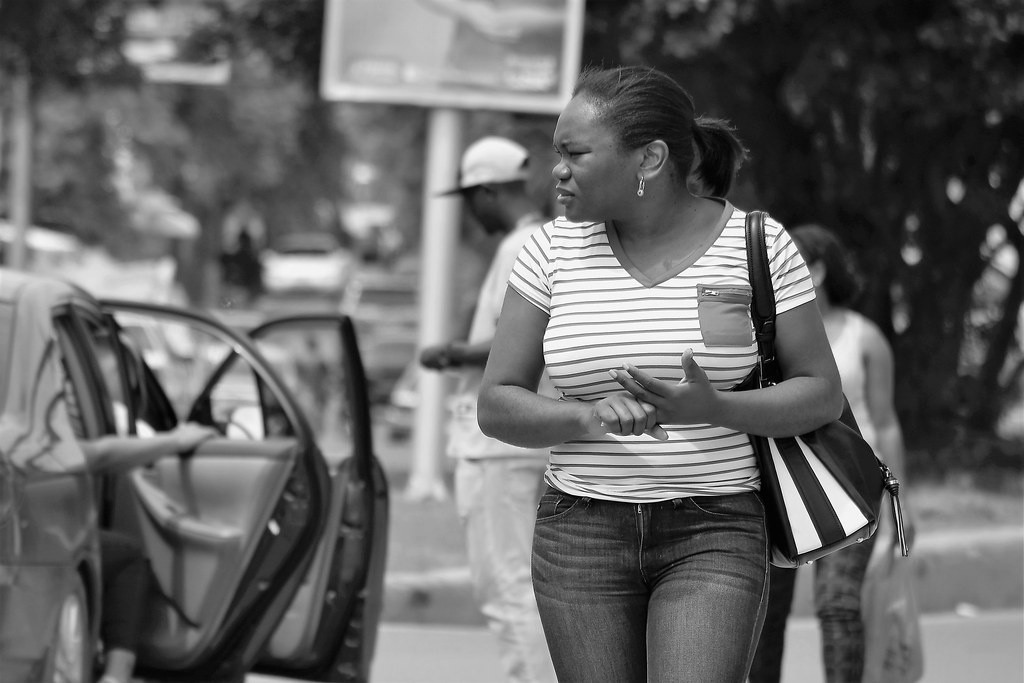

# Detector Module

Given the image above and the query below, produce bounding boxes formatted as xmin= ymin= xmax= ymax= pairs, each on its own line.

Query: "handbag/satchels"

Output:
xmin=735 ymin=211 xmax=887 ymax=569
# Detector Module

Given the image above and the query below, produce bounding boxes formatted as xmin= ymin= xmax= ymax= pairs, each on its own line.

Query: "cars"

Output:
xmin=0 ymin=265 xmax=392 ymax=681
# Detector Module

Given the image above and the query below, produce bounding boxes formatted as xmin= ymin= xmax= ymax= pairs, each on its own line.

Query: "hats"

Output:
xmin=435 ymin=135 xmax=532 ymax=196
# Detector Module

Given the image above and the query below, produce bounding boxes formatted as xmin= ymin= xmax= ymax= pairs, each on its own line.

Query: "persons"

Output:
xmin=473 ymin=60 xmax=844 ymax=683
xmin=416 ymin=136 xmax=564 ymax=683
xmin=750 ymin=228 xmax=914 ymax=683
xmin=70 ymin=422 xmax=223 ymax=683
